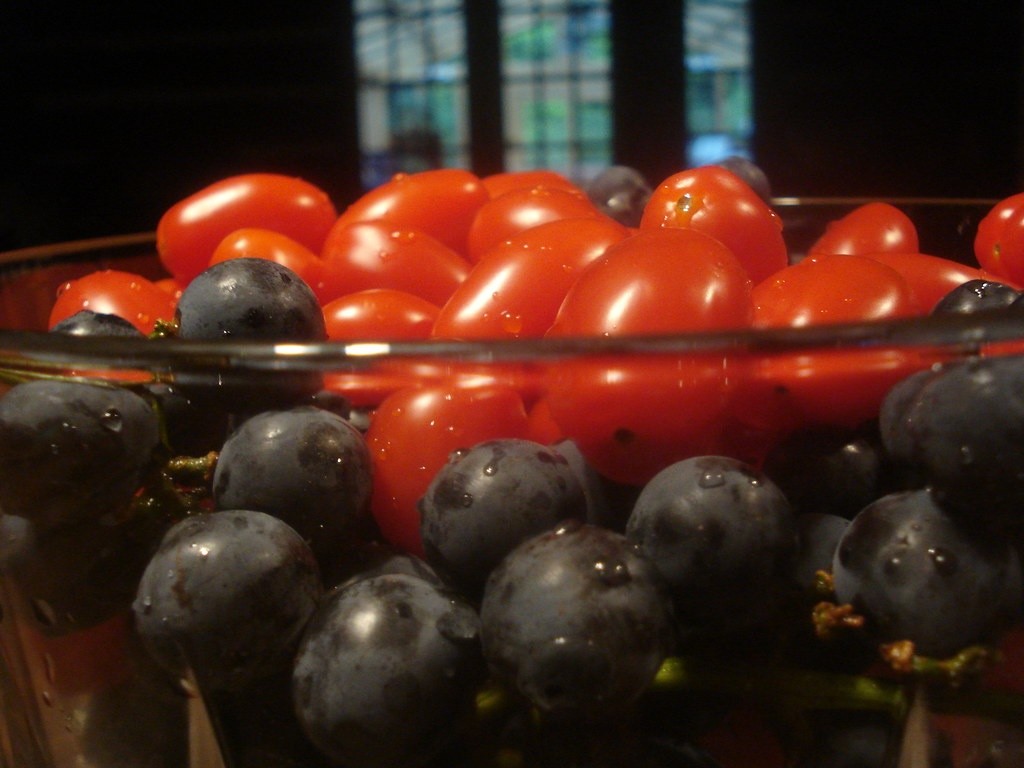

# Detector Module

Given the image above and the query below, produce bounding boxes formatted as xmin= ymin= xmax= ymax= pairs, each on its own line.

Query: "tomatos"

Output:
xmin=45 ymin=166 xmax=1024 ymax=561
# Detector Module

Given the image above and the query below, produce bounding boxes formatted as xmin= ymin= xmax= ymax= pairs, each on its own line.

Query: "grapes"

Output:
xmin=0 ymin=258 xmax=1024 ymax=768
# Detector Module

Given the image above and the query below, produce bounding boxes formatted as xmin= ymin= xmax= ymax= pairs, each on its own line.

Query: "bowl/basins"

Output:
xmin=0 ymin=198 xmax=1024 ymax=768
